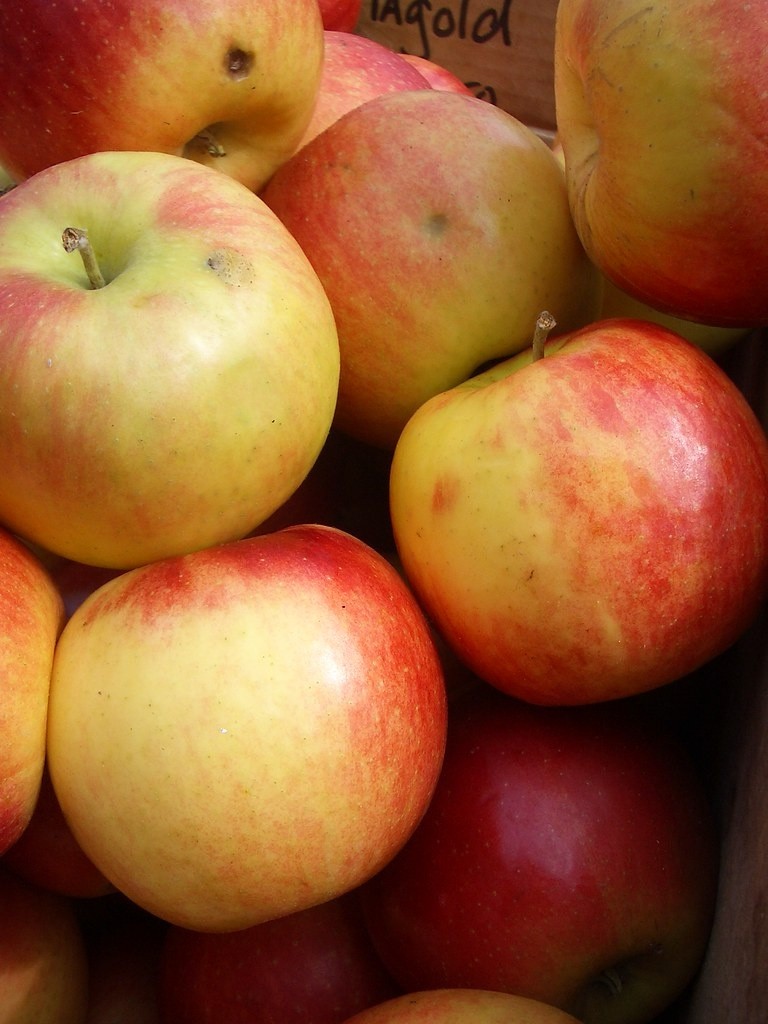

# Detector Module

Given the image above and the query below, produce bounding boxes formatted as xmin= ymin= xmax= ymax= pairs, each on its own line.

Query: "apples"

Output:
xmin=0 ymin=0 xmax=768 ymax=1024
